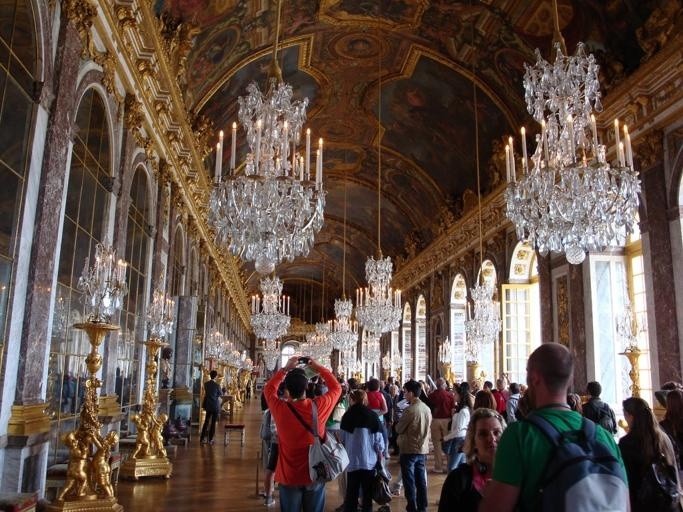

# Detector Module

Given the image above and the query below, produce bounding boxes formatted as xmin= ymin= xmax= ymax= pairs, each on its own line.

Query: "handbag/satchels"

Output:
xmin=649 ymin=458 xmax=679 ymax=510
xmin=260 ymin=411 xmax=272 ymax=440
xmin=373 ymin=472 xmax=392 ymax=505
xmin=442 ymin=439 xmax=455 ymax=455
xmin=309 ymin=431 xmax=350 ymax=482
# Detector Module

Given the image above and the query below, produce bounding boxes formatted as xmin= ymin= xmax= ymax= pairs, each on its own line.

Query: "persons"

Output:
xmin=130 ymin=413 xmax=168 ymax=459
xmin=58 ymin=430 xmax=117 ymax=502
xmin=200 ymin=370 xmax=223 ymax=445
xmin=55 ymin=366 xmax=128 ymax=413
xmin=393 ymin=83 xmax=488 ymax=150
xmin=246 ymin=382 xmax=251 ymax=399
xmin=160 ymin=348 xmax=173 ymax=369
xmin=262 ymin=342 xmax=682 ymax=511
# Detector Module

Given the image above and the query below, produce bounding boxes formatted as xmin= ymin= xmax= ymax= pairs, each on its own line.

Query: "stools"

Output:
xmin=224 ymin=425 xmax=246 ymax=447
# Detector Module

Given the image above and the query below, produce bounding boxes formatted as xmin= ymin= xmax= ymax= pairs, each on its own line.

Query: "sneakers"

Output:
xmin=391 ymin=483 xmax=401 ymax=496
xmin=358 ymin=504 xmax=372 ymax=512
xmin=264 ymin=496 xmax=275 ymax=506
xmin=432 ymin=466 xmax=443 ymax=473
xmin=336 ymin=503 xmax=358 ymax=512
xmin=378 ymin=504 xmax=391 ymax=512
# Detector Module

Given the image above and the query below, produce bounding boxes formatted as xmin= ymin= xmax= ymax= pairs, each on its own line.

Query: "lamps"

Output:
xmin=616 ymin=301 xmax=647 ymax=434
xmin=466 ymin=345 xmax=485 ymax=391
xmin=209 ymin=0 xmax=326 ymax=273
xmin=453 ymin=0 xmax=501 ymax=344
xmin=43 ymin=243 xmax=126 ymax=512
xmin=260 ymin=338 xmax=281 ymax=371
xmin=503 ymin=0 xmax=642 ymax=265
xmin=301 ymin=56 xmax=401 ymax=384
xmin=119 ymin=289 xmax=176 ymax=481
xmin=438 ymin=336 xmax=455 ymax=385
xmin=200 ymin=332 xmax=259 ymax=414
xmin=250 ymin=273 xmax=290 ymax=339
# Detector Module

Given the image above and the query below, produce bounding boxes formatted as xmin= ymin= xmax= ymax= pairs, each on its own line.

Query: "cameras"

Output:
xmin=298 ymin=357 xmax=308 ymax=364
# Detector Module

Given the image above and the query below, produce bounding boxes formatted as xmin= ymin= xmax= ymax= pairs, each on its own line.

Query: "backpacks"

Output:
xmin=525 ymin=411 xmax=632 ymax=512
xmin=588 ymin=401 xmax=617 ymax=435
